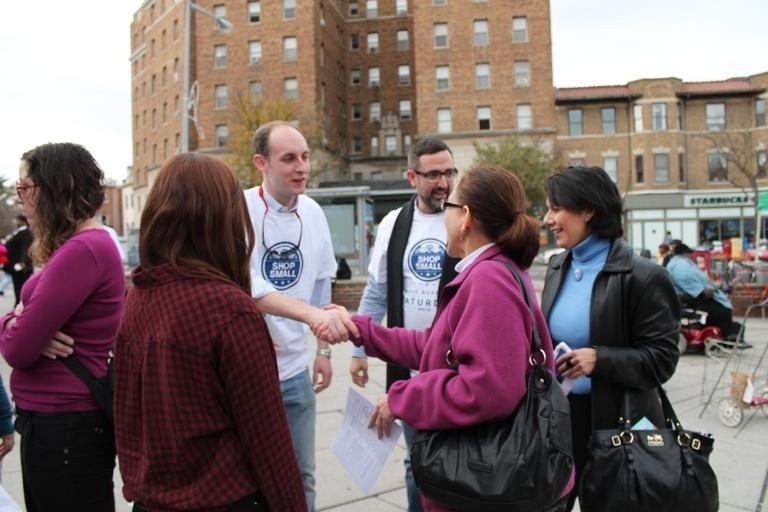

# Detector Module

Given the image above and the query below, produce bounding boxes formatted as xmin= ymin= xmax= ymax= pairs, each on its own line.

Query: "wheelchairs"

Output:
xmin=657 ymin=258 xmax=752 ymax=357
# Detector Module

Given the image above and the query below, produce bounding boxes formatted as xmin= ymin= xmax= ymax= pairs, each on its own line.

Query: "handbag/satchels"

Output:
xmin=573 ymin=419 xmax=719 ymax=512
xmin=94 ymin=355 xmax=117 ymax=420
xmin=403 ymin=364 xmax=576 ymax=512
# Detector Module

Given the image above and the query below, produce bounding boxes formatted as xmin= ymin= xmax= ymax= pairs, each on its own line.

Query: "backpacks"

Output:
xmin=336 ymin=259 xmax=351 ymax=281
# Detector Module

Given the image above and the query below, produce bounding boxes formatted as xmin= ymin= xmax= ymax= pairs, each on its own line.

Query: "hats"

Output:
xmin=674 ymin=244 xmax=694 ymax=256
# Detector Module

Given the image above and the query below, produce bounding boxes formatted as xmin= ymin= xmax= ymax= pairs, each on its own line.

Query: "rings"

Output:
xmin=566 ymin=359 xmax=574 ymax=368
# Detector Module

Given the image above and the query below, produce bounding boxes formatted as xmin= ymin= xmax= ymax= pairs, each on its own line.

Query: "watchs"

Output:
xmin=315 ymin=348 xmax=332 ymax=359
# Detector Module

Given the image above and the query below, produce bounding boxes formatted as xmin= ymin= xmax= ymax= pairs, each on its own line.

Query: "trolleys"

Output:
xmin=681 ymin=300 xmax=768 ymax=427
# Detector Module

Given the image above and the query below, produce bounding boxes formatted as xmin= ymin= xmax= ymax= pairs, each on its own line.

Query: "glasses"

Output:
xmin=438 ymin=198 xmax=478 ymax=221
xmin=411 ymin=165 xmax=460 ymax=181
xmin=15 ymin=180 xmax=44 ymax=197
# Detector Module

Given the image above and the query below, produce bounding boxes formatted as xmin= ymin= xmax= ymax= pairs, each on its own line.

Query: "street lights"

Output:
xmin=181 ymin=3 xmax=235 ymax=153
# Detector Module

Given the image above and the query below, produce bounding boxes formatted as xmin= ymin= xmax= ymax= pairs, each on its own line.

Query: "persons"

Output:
xmin=655 ymin=238 xmax=683 ymax=268
xmin=541 ymin=165 xmax=682 ymax=512
xmin=4 ymin=214 xmax=33 ymax=308
xmin=309 ymin=165 xmax=578 ymax=512
xmin=0 ymin=374 xmax=14 ymax=461
xmin=348 ymin=137 xmax=462 ymax=394
xmin=111 ymin=150 xmax=309 ymax=511
xmin=665 ymin=243 xmax=733 ymax=341
xmin=239 ymin=119 xmax=360 ymax=512
xmin=0 ymin=142 xmax=126 ymax=512
